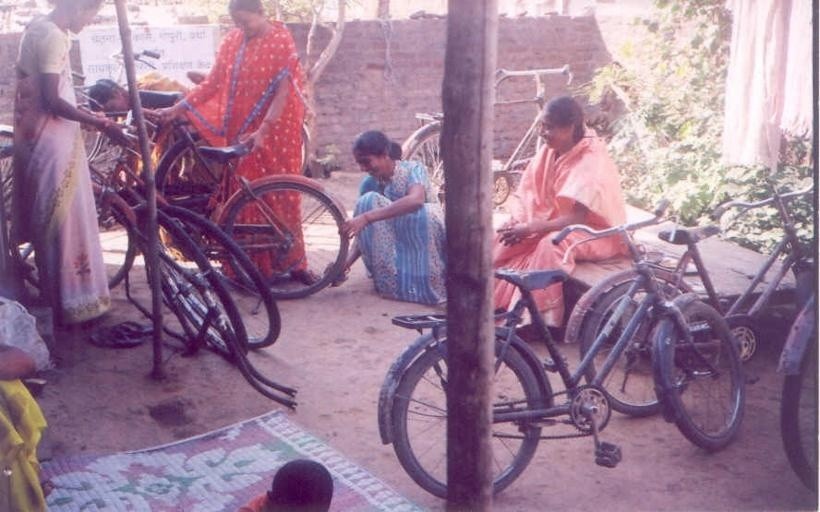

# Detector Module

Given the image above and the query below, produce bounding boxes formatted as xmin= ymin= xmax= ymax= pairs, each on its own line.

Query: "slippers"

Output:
xmin=113 ymin=321 xmax=155 ymax=337
xmin=92 ymin=329 xmax=142 ymax=349
xmin=291 ymin=269 xmax=320 ymax=285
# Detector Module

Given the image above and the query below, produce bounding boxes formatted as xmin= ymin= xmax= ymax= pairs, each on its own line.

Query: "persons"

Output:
xmin=87 ymin=77 xmax=216 ymax=262
xmin=323 ymin=130 xmax=450 ymax=303
xmin=0 ymin=287 xmax=50 ymax=512
xmin=13 ymin=0 xmax=138 ymax=327
xmin=494 ymin=95 xmax=637 ymax=333
xmin=236 ymin=455 xmax=336 ymax=511
xmin=154 ymin=0 xmax=320 ymax=288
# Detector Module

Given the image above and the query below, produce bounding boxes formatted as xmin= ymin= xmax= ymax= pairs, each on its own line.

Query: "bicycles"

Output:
xmin=84 ymin=116 xmax=352 ymax=302
xmin=401 ymin=64 xmax=578 ymax=208
xmin=0 ymin=118 xmax=280 ymax=360
xmin=376 ymin=195 xmax=744 ymax=501
xmin=771 ymin=289 xmax=817 ymax=486
xmin=564 ymin=194 xmax=817 ymax=419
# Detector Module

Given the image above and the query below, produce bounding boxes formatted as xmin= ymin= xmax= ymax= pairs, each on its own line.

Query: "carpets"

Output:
xmin=31 ymin=408 xmax=427 ymax=512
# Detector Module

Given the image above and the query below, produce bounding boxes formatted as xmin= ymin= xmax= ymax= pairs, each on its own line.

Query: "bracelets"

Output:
xmin=522 ymin=221 xmax=531 ymax=235
xmin=97 ymin=118 xmax=116 ymax=137
xmin=259 ymin=118 xmax=277 ymax=132
xmin=361 ymin=211 xmax=373 ymax=228
